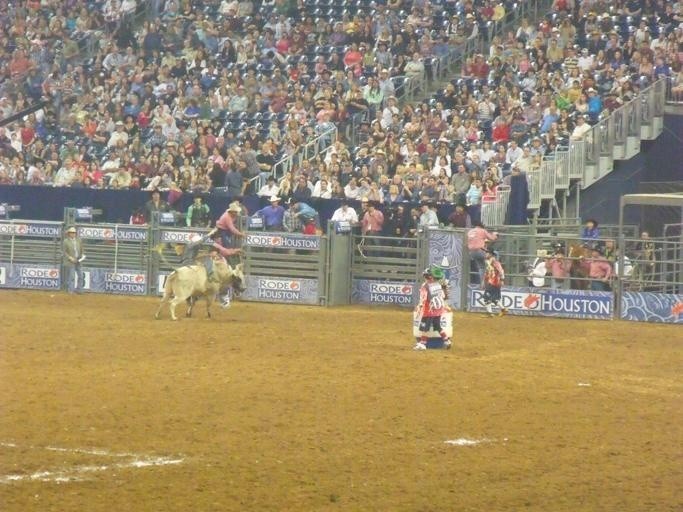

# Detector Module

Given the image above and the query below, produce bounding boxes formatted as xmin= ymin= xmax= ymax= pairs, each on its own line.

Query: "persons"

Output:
xmin=527 ymin=219 xmax=657 ymax=287
xmin=62 ymin=227 xmax=86 ymax=295
xmin=0 ymin=0 xmax=681 ymax=256
xmin=210 ymin=209 xmax=245 ymax=262
xmin=480 ymin=251 xmax=508 ymax=318
xmin=412 ymin=267 xmax=452 ymax=350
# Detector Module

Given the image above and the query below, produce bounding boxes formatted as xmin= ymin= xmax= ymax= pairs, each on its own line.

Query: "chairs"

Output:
xmin=0 ymin=0 xmax=682 ymax=206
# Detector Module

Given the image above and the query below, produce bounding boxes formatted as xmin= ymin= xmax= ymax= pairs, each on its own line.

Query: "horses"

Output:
xmin=566 ymin=243 xmax=590 ymax=290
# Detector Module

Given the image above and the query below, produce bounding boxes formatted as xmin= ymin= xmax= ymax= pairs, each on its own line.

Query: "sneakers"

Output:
xmin=412 ymin=341 xmax=427 ymax=352
xmin=444 ymin=340 xmax=452 ymax=348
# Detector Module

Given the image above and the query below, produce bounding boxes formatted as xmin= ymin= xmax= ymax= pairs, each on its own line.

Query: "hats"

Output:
xmin=266 ymin=195 xmax=282 ymax=203
xmin=226 ymin=203 xmax=241 ymax=211
xmin=479 ymin=247 xmax=496 ymax=255
xmin=429 ymin=267 xmax=443 ymax=279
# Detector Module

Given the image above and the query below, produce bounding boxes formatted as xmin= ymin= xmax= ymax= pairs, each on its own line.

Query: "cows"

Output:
xmin=150 ymin=242 xmax=247 ymax=321
